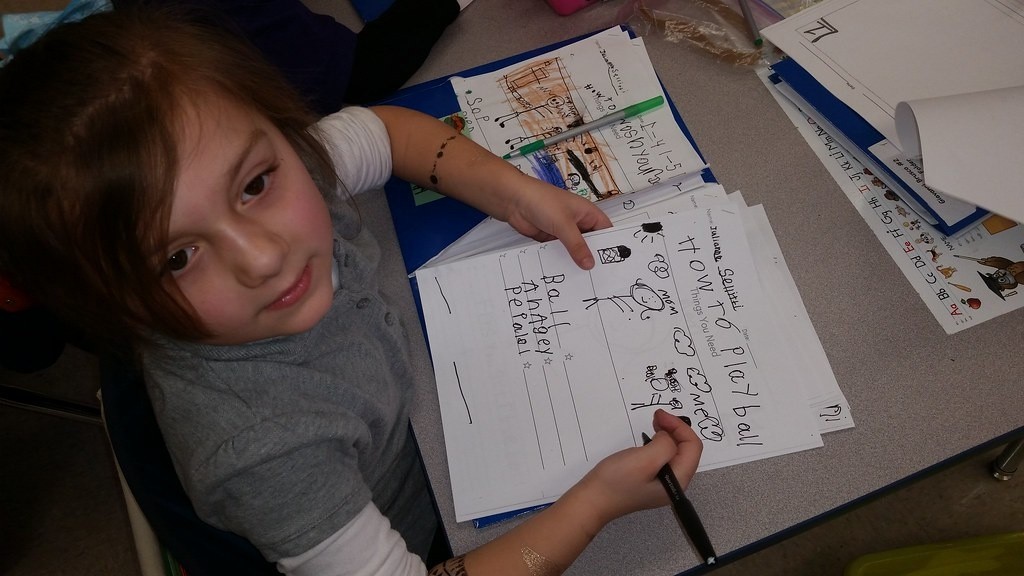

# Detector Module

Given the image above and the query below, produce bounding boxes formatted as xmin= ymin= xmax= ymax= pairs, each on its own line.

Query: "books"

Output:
xmin=757 ymin=0 xmax=1024 ymax=226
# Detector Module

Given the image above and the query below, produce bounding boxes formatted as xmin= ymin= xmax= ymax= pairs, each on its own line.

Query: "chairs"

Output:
xmin=98 ymin=297 xmax=280 ymax=576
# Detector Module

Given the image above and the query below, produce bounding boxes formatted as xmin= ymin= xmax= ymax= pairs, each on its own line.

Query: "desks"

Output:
xmin=349 ymin=1 xmax=1024 ymax=576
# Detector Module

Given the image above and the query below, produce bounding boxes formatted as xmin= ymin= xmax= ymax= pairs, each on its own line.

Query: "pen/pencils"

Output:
xmin=500 ymin=95 xmax=664 ymax=160
xmin=738 ymin=0 xmax=763 ymax=46
xmin=641 ymin=431 xmax=718 ymax=566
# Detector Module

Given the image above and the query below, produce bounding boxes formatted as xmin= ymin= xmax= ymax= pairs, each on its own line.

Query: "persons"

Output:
xmin=0 ymin=10 xmax=703 ymax=576
